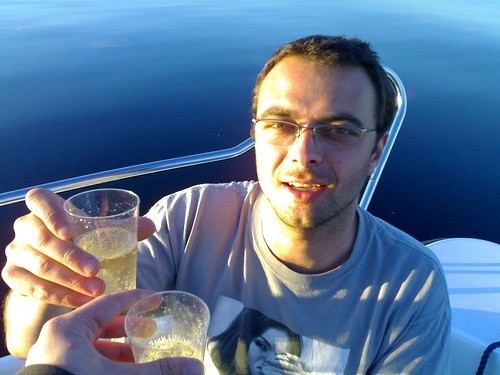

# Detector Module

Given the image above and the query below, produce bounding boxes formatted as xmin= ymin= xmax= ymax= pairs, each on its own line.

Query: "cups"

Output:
xmin=124 ymin=291 xmax=211 ymax=363
xmin=63 ymin=188 xmax=140 ymax=297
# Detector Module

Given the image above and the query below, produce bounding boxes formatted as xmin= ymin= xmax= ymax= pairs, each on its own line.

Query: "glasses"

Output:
xmin=251 ymin=119 xmax=378 ymax=150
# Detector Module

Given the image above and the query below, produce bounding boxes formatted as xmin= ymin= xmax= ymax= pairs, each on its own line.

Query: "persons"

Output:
xmin=1 ymin=33 xmax=454 ymax=374
